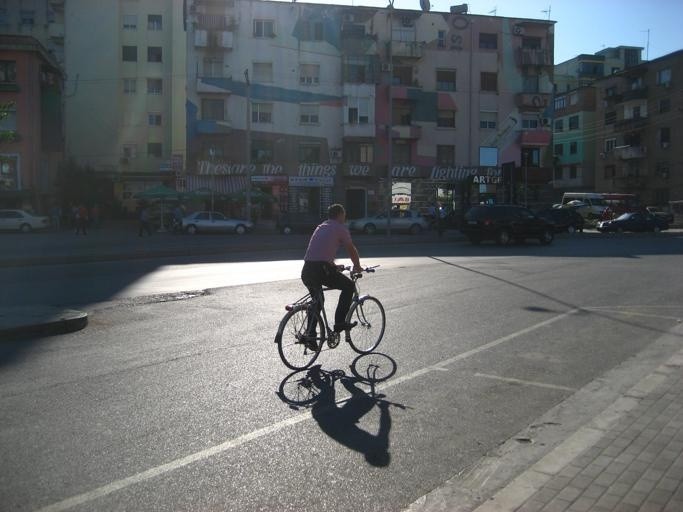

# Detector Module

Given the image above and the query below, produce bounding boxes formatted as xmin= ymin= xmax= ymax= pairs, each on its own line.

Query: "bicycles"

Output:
xmin=273 ymin=264 xmax=385 ymax=371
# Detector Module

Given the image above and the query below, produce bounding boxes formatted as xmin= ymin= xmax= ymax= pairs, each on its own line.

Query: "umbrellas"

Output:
xmin=226 ymin=187 xmax=279 ymax=217
xmin=179 ymin=186 xmax=228 ymax=211
xmin=564 ymin=200 xmax=587 ymax=214
xmin=132 ymin=185 xmax=185 ymax=233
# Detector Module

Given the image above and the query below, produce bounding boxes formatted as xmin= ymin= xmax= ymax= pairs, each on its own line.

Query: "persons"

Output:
xmin=51 ymin=202 xmax=61 ymax=234
xmin=74 ymin=206 xmax=87 ymax=236
xmin=427 ymin=202 xmax=435 ymax=219
xmin=138 ymin=205 xmax=152 ymax=237
xmin=602 ymin=207 xmax=611 ymax=220
xmin=171 ymin=203 xmax=182 ymax=223
xmin=299 ymin=203 xmax=363 ymax=352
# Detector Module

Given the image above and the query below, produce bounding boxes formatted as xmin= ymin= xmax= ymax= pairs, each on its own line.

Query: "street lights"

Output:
xmin=523 ymin=147 xmax=529 ymax=208
xmin=215 ymin=119 xmax=251 ymax=217
xmin=549 ymin=154 xmax=559 ymax=189
xmin=208 ymin=144 xmax=215 ymax=211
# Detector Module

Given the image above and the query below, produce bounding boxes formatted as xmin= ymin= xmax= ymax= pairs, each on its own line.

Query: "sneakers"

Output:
xmin=303 ymin=331 xmax=318 ymax=350
xmin=334 ymin=321 xmax=357 ymax=332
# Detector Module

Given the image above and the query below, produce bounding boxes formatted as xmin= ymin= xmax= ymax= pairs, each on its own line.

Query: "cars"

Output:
xmin=275 ymin=207 xmax=328 ymax=236
xmin=431 ymin=208 xmax=465 ymax=233
xmin=148 ymin=198 xmax=179 ymax=231
xmin=175 ymin=210 xmax=254 ymax=236
xmin=458 ymin=203 xmax=555 ymax=246
xmin=350 ymin=209 xmax=428 ymax=236
xmin=535 ymin=192 xmax=682 ymax=237
xmin=0 ymin=209 xmax=50 ymax=233
